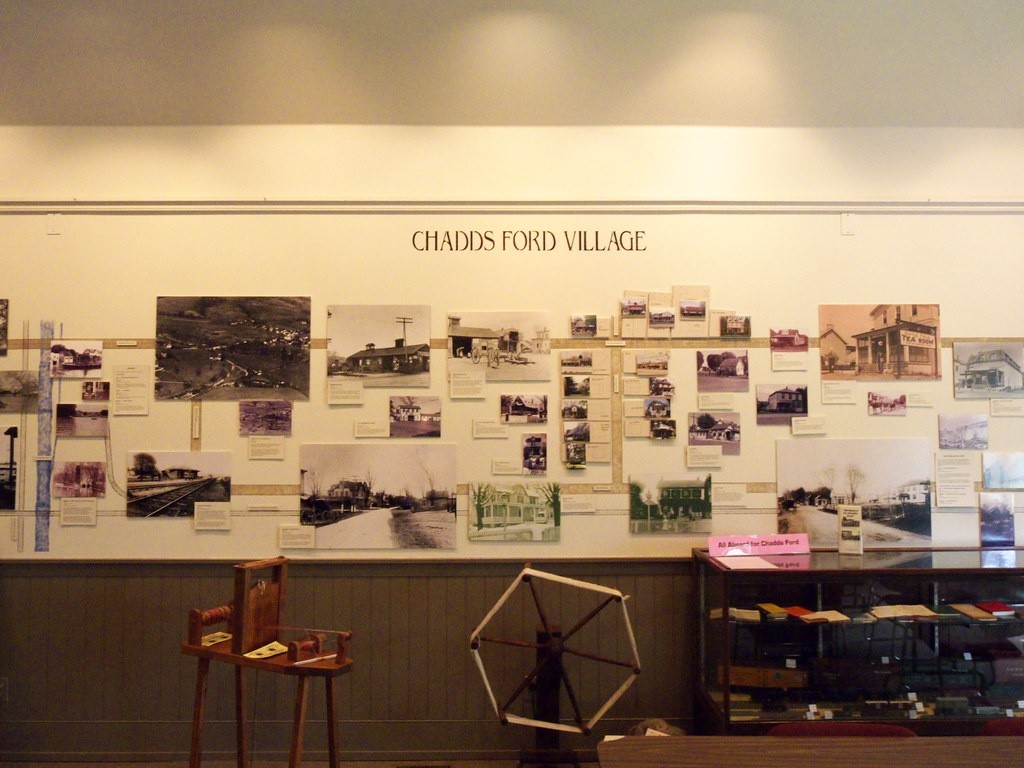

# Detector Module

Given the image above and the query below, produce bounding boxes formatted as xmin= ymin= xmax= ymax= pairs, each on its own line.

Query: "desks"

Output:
xmin=595 ymin=732 xmax=1024 ymax=768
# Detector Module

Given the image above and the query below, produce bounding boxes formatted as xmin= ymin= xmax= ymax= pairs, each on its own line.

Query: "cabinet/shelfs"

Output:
xmin=692 ymin=544 xmax=1024 ymax=738
